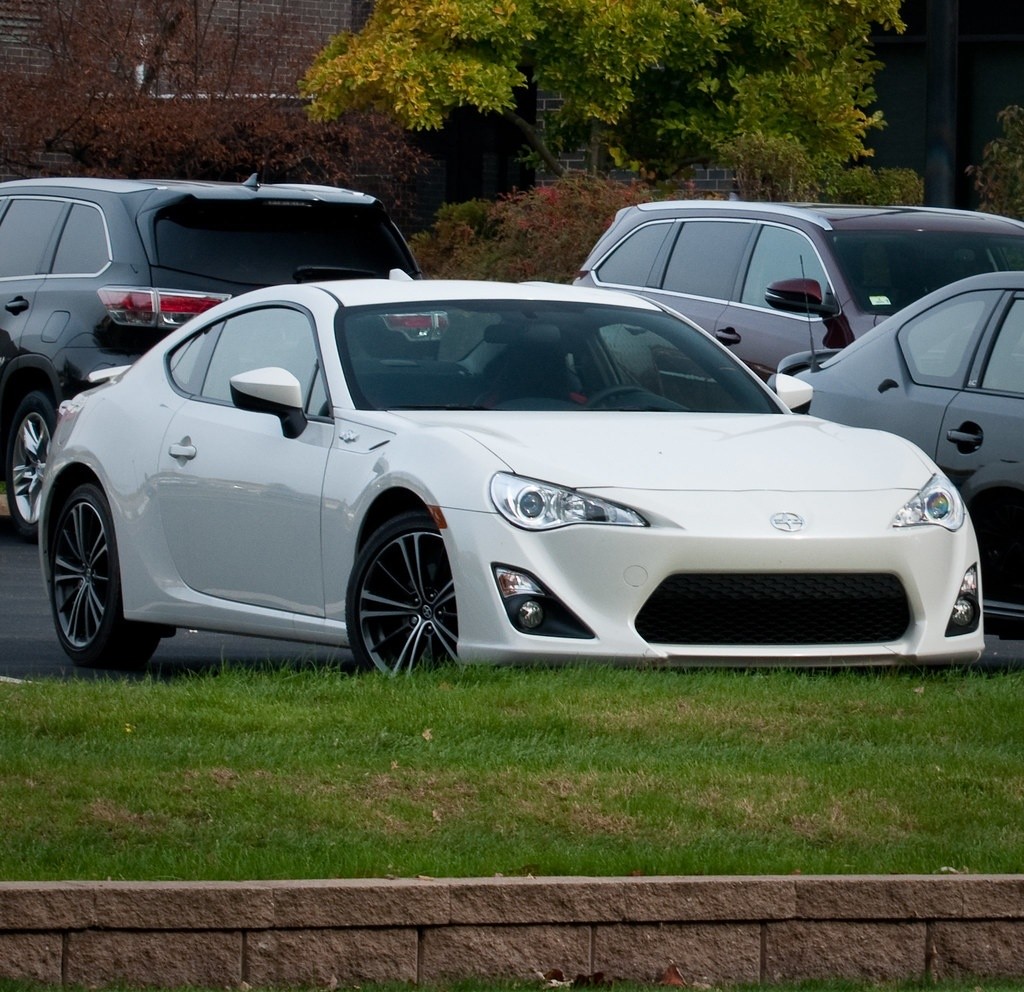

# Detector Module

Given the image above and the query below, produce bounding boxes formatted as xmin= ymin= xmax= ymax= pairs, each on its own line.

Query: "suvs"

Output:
xmin=0 ymin=172 xmax=448 ymax=544
xmin=570 ymin=196 xmax=1024 ymax=391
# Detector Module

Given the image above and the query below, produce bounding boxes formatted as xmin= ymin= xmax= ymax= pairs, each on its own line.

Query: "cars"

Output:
xmin=766 ymin=269 xmax=1023 ymax=643
xmin=33 ymin=268 xmax=987 ymax=681
